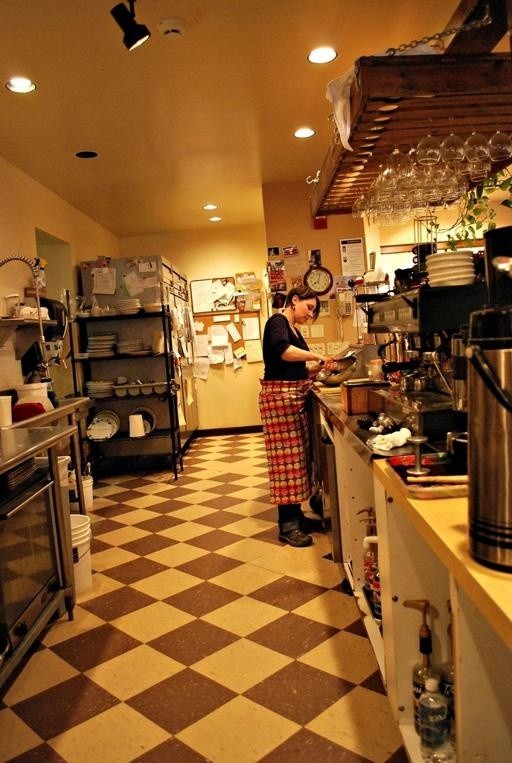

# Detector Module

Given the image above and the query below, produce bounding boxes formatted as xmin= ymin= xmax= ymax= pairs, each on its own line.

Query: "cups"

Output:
xmin=6 ymin=296 xmax=22 ymax=318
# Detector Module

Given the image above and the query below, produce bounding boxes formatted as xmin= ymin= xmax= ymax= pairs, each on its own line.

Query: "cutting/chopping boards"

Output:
xmin=313 ymin=378 xmax=371 ymax=394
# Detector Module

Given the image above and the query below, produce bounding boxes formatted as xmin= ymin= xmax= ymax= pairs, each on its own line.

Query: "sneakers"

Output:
xmin=279 ymin=528 xmax=312 ymax=546
xmin=300 ymin=518 xmax=322 ymax=530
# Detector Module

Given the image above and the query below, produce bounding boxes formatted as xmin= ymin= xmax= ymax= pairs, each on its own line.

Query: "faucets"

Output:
xmin=48 ymin=356 xmax=67 ymax=370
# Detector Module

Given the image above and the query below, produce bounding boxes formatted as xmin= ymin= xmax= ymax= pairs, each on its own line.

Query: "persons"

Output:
xmin=259 ymin=287 xmax=335 ymax=546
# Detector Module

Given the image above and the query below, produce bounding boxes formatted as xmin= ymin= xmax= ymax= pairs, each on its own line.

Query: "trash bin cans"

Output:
xmin=34 ymin=456 xmax=75 ymax=619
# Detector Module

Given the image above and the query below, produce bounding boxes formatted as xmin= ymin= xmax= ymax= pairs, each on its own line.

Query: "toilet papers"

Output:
xmin=129 ymin=414 xmax=145 ymax=438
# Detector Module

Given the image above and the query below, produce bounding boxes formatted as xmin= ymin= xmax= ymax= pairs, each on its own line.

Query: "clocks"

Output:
xmin=303 ymin=267 xmax=333 ymax=295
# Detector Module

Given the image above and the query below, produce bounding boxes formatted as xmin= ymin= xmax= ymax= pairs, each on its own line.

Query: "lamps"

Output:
xmin=110 ymin=0 xmax=149 ymax=51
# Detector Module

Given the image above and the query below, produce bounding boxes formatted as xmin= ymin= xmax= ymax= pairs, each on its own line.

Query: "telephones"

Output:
xmin=338 ymin=291 xmax=353 ymax=316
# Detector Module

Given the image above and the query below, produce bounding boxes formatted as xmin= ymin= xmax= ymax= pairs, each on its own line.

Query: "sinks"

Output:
xmin=34 ymin=455 xmax=71 ymax=472
xmin=0 ymin=397 xmax=89 ymax=428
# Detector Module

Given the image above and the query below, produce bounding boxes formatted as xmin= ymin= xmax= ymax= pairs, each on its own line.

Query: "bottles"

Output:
xmin=362 ymin=322 xmax=376 ymax=345
xmin=467 ymin=304 xmax=512 ymax=573
xmin=403 ymin=626 xmax=455 ymax=763
xmin=356 ymin=521 xmax=382 ymax=636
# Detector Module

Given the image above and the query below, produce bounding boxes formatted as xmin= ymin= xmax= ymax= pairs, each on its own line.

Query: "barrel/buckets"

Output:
xmin=15 ymin=383 xmax=48 ymax=401
xmin=75 ymin=475 xmax=94 ymax=511
xmin=69 ymin=514 xmax=93 ymax=595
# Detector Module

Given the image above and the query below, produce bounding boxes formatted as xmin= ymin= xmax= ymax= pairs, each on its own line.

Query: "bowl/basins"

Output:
xmin=315 ymin=355 xmax=358 ymax=386
xmin=425 ymin=250 xmax=476 ymax=286
xmin=143 ymin=303 xmax=164 ymax=312
xmin=116 ymin=299 xmax=142 ymax=315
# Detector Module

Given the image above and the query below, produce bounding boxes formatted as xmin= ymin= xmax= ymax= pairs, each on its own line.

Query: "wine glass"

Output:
xmin=351 ymin=115 xmax=512 ymax=229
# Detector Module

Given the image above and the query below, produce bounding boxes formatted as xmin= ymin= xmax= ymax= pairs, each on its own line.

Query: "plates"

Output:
xmin=85 ymin=381 xmax=113 ymax=398
xmin=117 ymin=337 xmax=144 ymax=354
xmin=86 ymin=406 xmax=157 ymax=442
xmin=127 ymin=350 xmax=151 ymax=355
xmin=85 ymin=334 xmax=116 ymax=357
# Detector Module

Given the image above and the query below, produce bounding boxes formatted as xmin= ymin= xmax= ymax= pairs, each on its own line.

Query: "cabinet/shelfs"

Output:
xmin=76 ymin=282 xmax=184 ymax=481
